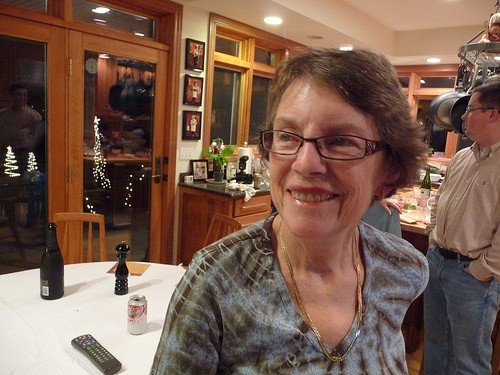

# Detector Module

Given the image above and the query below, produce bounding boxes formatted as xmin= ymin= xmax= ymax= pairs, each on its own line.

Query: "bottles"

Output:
xmin=212 ymin=147 xmax=220 ymax=177
xmin=420 ymin=167 xmax=431 ymax=199
xmin=40 ymin=222 xmax=64 ymax=301
xmin=115 ymin=240 xmax=131 ymax=294
xmin=428 ymin=144 xmax=432 ymax=157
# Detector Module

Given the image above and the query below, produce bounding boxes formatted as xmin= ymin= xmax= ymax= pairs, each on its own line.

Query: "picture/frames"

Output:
xmin=190 ymin=159 xmax=209 ymax=181
xmin=184 ymin=74 xmax=204 ymax=106
xmin=185 ymin=38 xmax=206 ymax=72
xmin=181 ymin=110 xmax=202 ymax=141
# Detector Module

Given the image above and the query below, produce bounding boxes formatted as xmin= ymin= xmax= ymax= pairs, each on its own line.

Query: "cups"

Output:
xmin=427 ymin=197 xmax=436 ymax=222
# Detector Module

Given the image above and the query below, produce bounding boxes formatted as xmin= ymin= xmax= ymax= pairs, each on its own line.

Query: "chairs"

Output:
xmin=51 ymin=211 xmax=107 ymax=262
xmin=201 ymin=214 xmax=242 ymax=250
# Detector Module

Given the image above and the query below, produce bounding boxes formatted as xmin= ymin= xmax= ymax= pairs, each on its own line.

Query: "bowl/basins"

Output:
xmin=420 ymin=173 xmax=442 ymax=182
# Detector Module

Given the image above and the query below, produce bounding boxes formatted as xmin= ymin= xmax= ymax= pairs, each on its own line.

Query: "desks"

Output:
xmin=0 ymin=261 xmax=187 ymax=375
xmin=389 ymin=182 xmax=440 ymax=235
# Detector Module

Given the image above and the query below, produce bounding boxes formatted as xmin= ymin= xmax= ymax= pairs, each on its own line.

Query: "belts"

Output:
xmin=439 ymin=245 xmax=476 ymax=261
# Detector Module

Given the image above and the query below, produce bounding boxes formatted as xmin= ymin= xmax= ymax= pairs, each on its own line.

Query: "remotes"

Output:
xmin=71 ymin=334 xmax=121 ymax=375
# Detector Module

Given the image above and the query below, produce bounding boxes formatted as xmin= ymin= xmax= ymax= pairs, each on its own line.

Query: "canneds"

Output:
xmin=127 ymin=295 xmax=148 ymax=334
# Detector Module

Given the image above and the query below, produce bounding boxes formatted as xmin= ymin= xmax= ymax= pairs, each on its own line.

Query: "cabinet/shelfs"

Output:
xmin=178 ymin=186 xmax=272 ymax=266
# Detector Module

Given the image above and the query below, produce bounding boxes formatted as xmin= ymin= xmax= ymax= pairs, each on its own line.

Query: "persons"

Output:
xmin=148 ymin=49 xmax=430 ymax=374
xmin=376 ymin=197 xmax=405 ymax=215
xmin=187 ymin=45 xmax=203 ymax=134
xmin=418 ymin=81 xmax=500 ymax=375
xmin=0 ymin=82 xmax=45 ymax=226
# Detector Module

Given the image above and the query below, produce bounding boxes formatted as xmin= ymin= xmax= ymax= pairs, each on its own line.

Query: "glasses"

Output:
xmin=465 ymin=106 xmax=500 ymax=115
xmin=259 ymin=129 xmax=388 ymax=161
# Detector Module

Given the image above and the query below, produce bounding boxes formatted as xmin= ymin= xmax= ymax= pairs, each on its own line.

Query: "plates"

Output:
xmin=386 ymin=199 xmax=415 ymax=213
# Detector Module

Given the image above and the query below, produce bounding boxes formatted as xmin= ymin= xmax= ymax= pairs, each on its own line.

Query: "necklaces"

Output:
xmin=279 ymin=218 xmax=363 ymax=364
